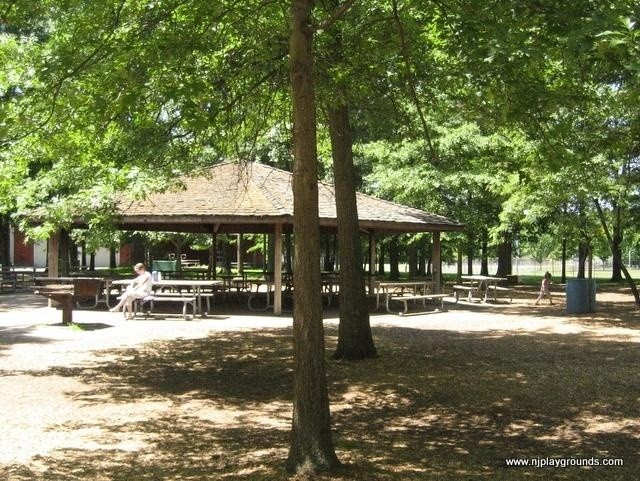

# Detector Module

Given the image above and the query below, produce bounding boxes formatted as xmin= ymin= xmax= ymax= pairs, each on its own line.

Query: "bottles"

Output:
xmin=158 ymin=272 xmax=162 ymax=283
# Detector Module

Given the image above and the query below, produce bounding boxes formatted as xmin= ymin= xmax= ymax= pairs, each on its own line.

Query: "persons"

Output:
xmin=534 ymin=271 xmax=561 ymax=306
xmin=109 ymin=262 xmax=153 ymax=322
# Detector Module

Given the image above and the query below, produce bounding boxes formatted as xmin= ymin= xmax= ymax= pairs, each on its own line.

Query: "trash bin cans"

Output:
xmin=566 ymin=278 xmax=596 ymax=314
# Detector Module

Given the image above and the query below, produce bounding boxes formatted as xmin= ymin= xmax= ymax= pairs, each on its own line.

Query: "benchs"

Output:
xmin=0 ymin=260 xmax=507 ymax=325
